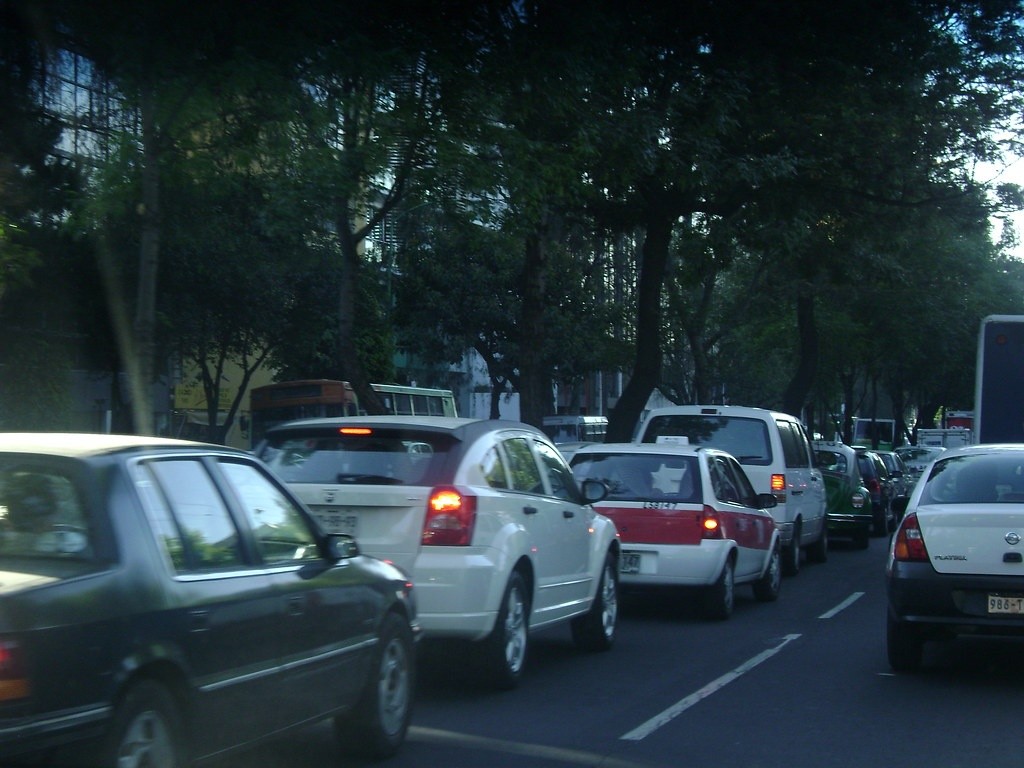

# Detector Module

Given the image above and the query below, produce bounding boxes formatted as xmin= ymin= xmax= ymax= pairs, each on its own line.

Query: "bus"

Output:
xmin=542 ymin=414 xmax=609 ymax=445
xmin=237 ymin=379 xmax=459 ymax=450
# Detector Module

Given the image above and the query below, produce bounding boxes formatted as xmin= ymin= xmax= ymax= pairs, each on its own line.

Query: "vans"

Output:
xmin=634 ymin=404 xmax=838 ymax=578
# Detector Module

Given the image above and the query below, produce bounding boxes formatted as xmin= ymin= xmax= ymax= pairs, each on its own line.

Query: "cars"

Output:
xmin=561 ymin=441 xmax=783 ymax=621
xmin=401 ymin=441 xmax=434 ymax=464
xmin=806 ymin=438 xmax=877 ymax=542
xmin=842 ymin=314 xmax=1024 ymax=543
xmin=884 ymin=443 xmax=1024 ymax=675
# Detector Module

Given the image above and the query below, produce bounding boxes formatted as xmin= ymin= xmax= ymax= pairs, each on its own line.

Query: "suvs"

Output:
xmin=0 ymin=427 xmax=424 ymax=768
xmin=230 ymin=414 xmax=625 ymax=692
